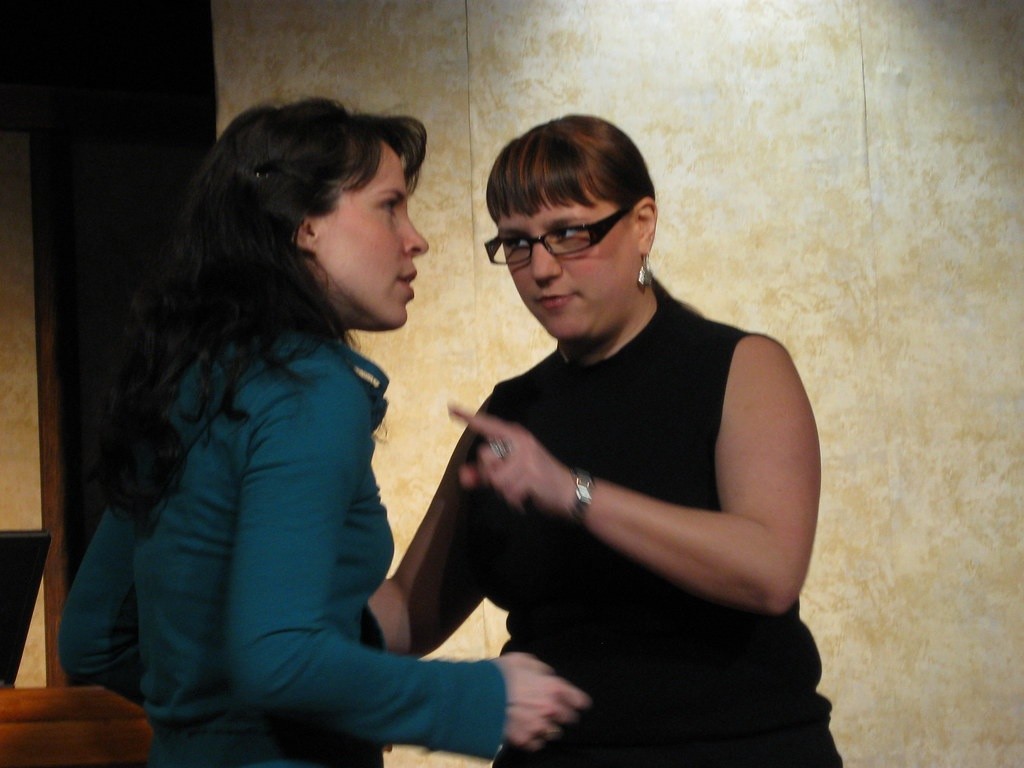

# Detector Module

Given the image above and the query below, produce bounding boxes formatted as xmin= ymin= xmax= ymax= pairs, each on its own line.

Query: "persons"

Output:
xmin=365 ymin=115 xmax=836 ymax=768
xmin=52 ymin=95 xmax=591 ymax=768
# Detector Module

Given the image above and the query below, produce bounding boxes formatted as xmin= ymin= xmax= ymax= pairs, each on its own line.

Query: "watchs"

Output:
xmin=569 ymin=466 xmax=594 ymax=524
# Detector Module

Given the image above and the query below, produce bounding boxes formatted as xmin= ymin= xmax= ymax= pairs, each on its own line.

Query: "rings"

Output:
xmin=490 ymin=438 xmax=508 ymax=460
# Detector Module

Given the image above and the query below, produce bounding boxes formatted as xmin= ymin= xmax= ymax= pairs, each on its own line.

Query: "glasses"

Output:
xmin=484 ymin=208 xmax=630 ymax=265
